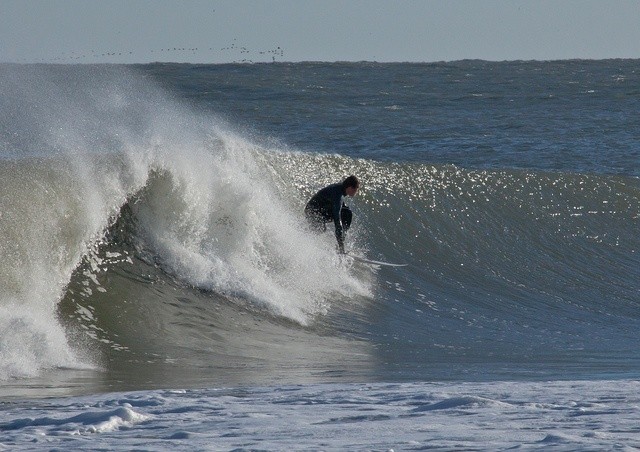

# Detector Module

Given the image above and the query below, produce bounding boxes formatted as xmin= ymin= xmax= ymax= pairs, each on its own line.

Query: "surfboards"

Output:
xmin=334 ymin=248 xmax=409 ymax=268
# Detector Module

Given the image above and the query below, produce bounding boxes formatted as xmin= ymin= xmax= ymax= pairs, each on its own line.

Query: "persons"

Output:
xmin=304 ymin=175 xmax=360 ymax=254
